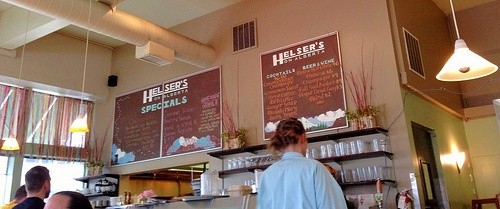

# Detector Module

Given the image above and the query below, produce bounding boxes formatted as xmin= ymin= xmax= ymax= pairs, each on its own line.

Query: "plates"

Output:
xmin=200 ymin=173 xmax=219 ymax=197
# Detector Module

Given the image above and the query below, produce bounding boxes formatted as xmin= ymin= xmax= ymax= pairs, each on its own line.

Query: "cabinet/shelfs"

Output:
xmin=207 ymin=127 xmax=396 ymax=197
xmin=76 ymin=174 xmax=120 ymax=198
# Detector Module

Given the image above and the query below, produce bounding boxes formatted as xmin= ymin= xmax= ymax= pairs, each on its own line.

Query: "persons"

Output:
xmin=0 ymin=183 xmax=27 ymax=209
xmin=257 ymin=117 xmax=349 ymax=209
xmin=11 ymin=166 xmax=51 ymax=209
xmin=43 ymin=191 xmax=93 ymax=209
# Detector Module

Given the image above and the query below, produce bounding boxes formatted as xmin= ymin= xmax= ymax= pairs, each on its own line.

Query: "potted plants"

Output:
xmin=219 ymin=105 xmax=247 ymax=149
xmin=86 ymin=128 xmax=108 ymax=176
xmin=343 ymin=42 xmax=382 ymax=130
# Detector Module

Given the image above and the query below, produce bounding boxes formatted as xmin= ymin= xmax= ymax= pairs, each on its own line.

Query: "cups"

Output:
xmin=88 ymin=197 xmax=120 ymax=209
xmin=244 ymin=178 xmax=257 ymax=192
xmin=306 ymin=137 xmax=390 ymax=158
xmin=191 ymin=179 xmax=200 ymax=196
xmin=230 ymin=152 xmax=282 ymax=169
xmin=339 ymin=165 xmax=393 ymax=182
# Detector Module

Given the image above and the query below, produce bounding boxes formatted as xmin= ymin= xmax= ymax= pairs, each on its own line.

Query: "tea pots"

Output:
xmin=77 ymin=180 xmax=118 ymax=195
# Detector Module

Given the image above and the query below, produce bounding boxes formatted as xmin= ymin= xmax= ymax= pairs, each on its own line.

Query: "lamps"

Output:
xmin=69 ymin=0 xmax=92 ymax=133
xmin=436 ymin=0 xmax=498 ymax=82
xmin=2 ymin=10 xmax=30 ymax=151
